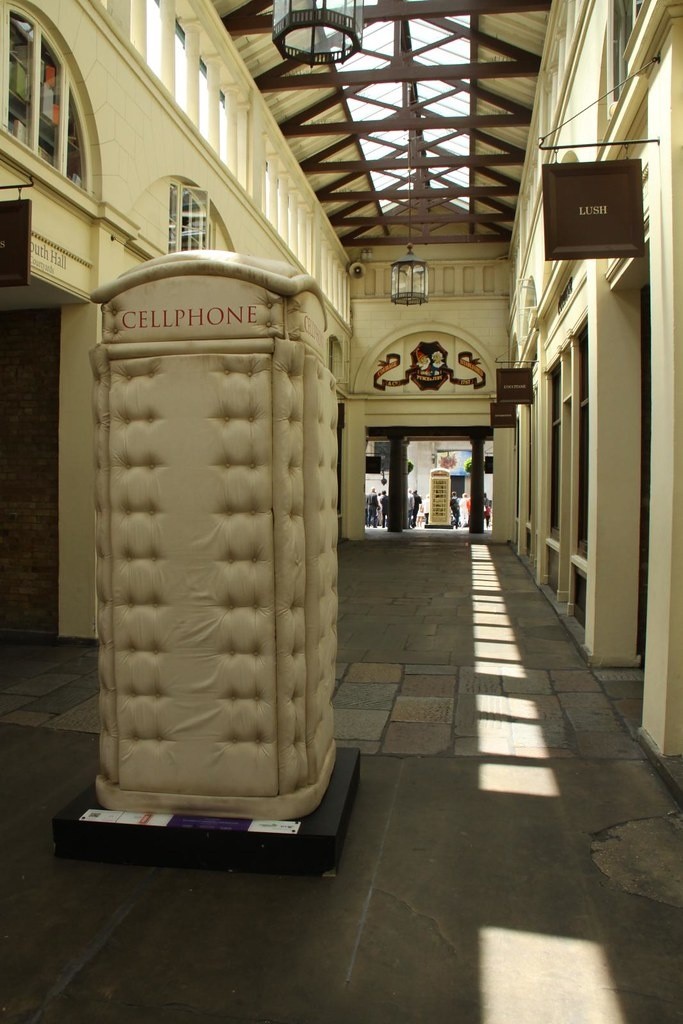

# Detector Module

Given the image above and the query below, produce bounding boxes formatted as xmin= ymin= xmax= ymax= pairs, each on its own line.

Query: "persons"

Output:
xmin=376 ymin=493 xmax=382 ymax=526
xmin=423 ymin=496 xmax=430 ymax=524
xmin=408 ymin=489 xmax=414 ymax=529
xmin=417 ymin=495 xmax=424 ymax=528
xmin=459 ymin=493 xmax=469 ymax=528
xmin=412 ymin=490 xmax=422 ymax=528
xmin=483 ymin=492 xmax=491 ymax=527
xmin=380 ymin=491 xmax=388 ymax=528
xmin=449 ymin=491 xmax=461 ymax=529
xmin=366 ymin=487 xmax=379 ymax=528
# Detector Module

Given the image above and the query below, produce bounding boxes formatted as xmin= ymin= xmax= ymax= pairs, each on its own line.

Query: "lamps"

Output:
xmin=271 ymin=0 xmax=366 ymax=69
xmin=385 ymin=138 xmax=429 ymax=307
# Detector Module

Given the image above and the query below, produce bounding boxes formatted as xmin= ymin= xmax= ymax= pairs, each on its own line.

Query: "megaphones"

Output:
xmin=349 ymin=262 xmax=366 ymax=279
